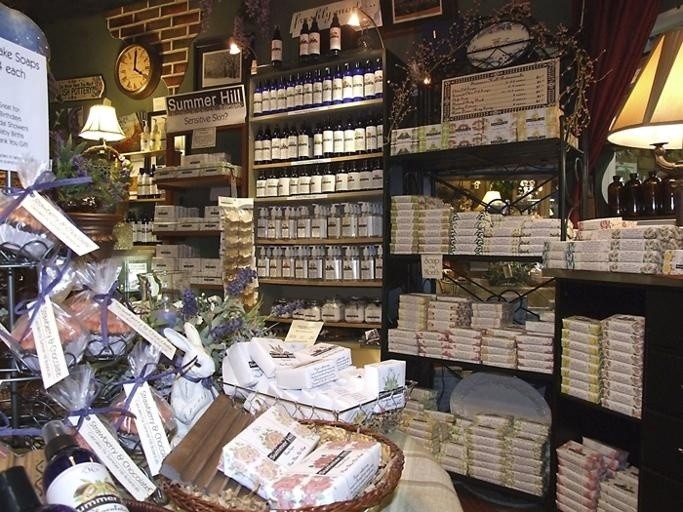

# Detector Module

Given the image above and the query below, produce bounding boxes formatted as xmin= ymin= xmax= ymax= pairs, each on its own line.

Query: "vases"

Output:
xmin=61 ymin=211 xmax=123 ymax=263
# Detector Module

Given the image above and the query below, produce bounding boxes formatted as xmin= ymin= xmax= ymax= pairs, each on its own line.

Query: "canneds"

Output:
xmin=270 ymin=295 xmax=382 ymax=324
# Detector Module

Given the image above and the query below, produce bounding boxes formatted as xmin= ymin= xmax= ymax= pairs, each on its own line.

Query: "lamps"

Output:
xmin=77 ymin=104 xmax=130 ymax=154
xmin=227 ymin=35 xmax=257 ymax=61
xmin=344 ymin=6 xmax=388 ymax=54
xmin=604 ymin=22 xmax=681 ymax=226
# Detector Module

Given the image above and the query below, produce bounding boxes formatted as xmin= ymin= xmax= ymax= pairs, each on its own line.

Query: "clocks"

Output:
xmin=111 ymin=40 xmax=162 ymax=101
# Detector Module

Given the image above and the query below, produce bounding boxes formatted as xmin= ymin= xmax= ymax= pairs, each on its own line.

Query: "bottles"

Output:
xmin=247 ymin=13 xmax=383 ymax=197
xmin=123 ymin=161 xmax=160 ymax=248
xmin=604 ymin=170 xmax=682 ymax=226
xmin=0 ymin=464 xmax=78 ymax=512
xmin=39 ymin=418 xmax=130 ymax=511
xmin=286 ymin=294 xmax=381 ymax=324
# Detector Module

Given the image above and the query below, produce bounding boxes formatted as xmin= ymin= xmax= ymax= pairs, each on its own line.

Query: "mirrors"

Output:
xmin=430 ymin=172 xmax=555 ymax=325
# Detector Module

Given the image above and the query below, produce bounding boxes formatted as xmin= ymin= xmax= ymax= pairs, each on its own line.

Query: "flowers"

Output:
xmin=164 ymin=264 xmax=305 ymax=380
xmin=49 ymin=129 xmax=136 ymax=216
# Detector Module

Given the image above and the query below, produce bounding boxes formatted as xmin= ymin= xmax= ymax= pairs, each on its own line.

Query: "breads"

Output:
xmin=0 ymin=197 xmax=60 ymax=242
xmin=110 ymin=386 xmax=172 ymax=434
xmin=67 ymin=425 xmax=93 ymax=454
xmin=10 ymin=298 xmax=135 ymax=355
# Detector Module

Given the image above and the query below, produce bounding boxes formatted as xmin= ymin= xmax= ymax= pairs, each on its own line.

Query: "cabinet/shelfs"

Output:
xmin=250 ymin=46 xmax=410 ymax=353
xmin=384 ymin=138 xmax=586 ymax=510
xmin=120 ymin=146 xmax=168 ymax=251
xmin=150 ymin=164 xmax=248 ymax=295
xmin=537 ymin=264 xmax=682 ymax=512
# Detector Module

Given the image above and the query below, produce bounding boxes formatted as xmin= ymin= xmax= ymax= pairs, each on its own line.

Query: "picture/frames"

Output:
xmin=387 ymin=0 xmax=447 ymax=30
xmin=192 ymin=43 xmax=248 ymax=93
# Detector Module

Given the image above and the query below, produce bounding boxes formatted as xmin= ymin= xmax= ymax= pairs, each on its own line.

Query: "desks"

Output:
xmin=2 ymin=416 xmax=467 ymax=512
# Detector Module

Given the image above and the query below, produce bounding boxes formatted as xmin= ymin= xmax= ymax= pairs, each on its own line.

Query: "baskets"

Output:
xmin=157 ymin=414 xmax=407 ymax=512
